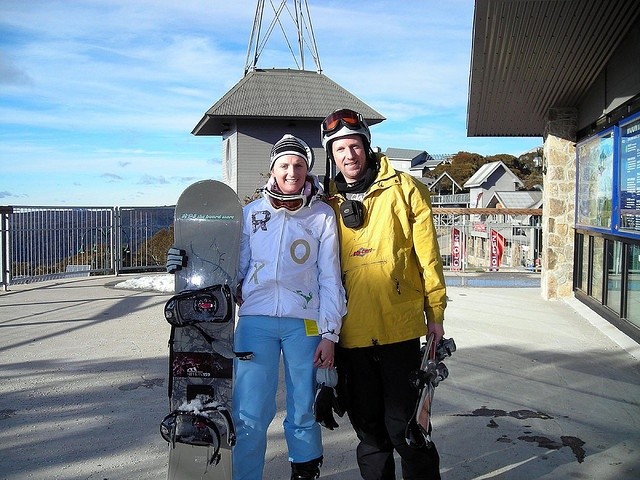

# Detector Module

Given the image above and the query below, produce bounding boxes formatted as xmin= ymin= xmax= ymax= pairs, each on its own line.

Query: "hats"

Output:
xmin=268 ymin=134 xmax=309 ymax=174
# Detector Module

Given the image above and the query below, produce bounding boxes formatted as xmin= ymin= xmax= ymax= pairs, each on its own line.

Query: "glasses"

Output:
xmin=321 ymin=109 xmax=371 ymax=139
xmin=266 ymin=189 xmax=307 ymax=216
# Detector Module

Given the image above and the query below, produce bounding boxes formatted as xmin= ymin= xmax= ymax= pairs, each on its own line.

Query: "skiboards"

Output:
xmin=404 ymin=330 xmax=456 ymax=450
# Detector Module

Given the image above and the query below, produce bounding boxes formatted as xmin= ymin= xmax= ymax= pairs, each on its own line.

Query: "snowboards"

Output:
xmin=161 ymin=178 xmax=242 ymax=480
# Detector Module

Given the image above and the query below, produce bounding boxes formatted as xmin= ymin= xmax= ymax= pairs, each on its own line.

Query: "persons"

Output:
xmin=313 ymin=108 xmax=446 ymax=480
xmin=166 ymin=133 xmax=349 ymax=480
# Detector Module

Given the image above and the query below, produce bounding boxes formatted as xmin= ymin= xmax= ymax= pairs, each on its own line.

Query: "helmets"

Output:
xmin=322 ymin=109 xmax=374 ymax=158
xmin=314 ymin=381 xmax=345 ymax=430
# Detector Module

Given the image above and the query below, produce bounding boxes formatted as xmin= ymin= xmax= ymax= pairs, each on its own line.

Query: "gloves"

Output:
xmin=165 ymin=247 xmax=189 ymax=274
